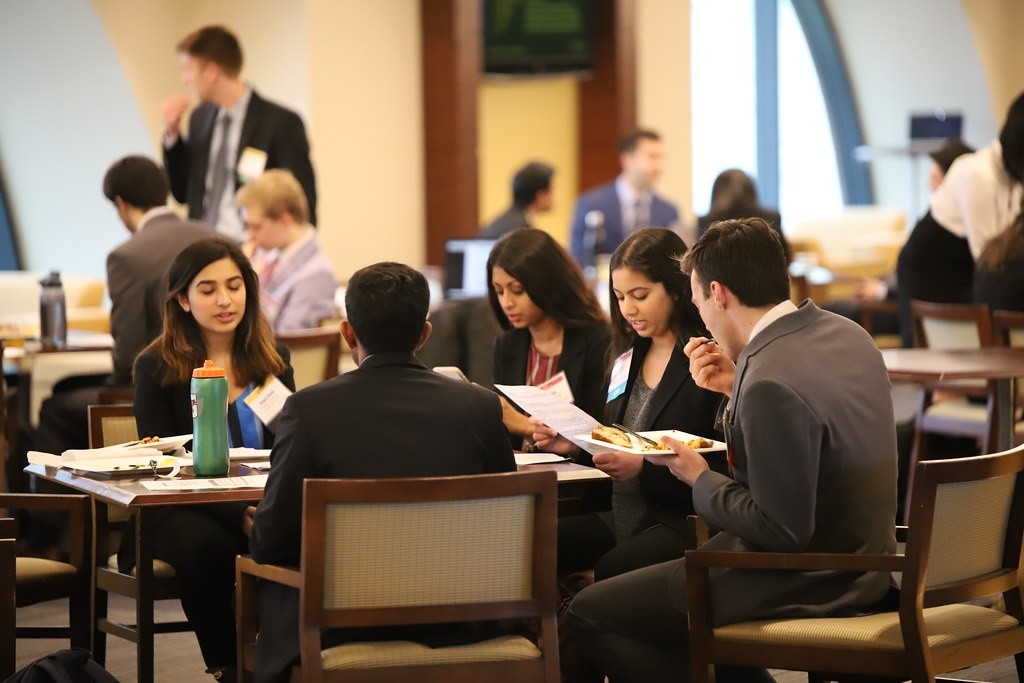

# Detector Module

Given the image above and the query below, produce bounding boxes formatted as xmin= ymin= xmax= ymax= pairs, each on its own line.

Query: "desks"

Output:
xmin=22 ymin=454 xmax=609 ymax=683
xmin=873 ymin=345 xmax=1024 ymax=488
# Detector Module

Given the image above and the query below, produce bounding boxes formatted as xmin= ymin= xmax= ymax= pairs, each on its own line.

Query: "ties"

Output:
xmin=633 ymin=199 xmax=643 ymax=232
xmin=202 ymin=116 xmax=233 ymax=228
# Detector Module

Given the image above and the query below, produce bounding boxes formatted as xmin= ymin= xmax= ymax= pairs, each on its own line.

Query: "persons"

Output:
xmin=819 ymin=139 xmax=975 ymax=336
xmin=472 ymin=229 xmax=611 ymax=517
xmin=695 ymin=169 xmax=792 ymax=267
xmin=477 ymin=161 xmax=556 ymax=240
xmin=568 ymin=126 xmax=680 ymax=292
xmin=238 ymin=169 xmax=343 ymax=332
xmin=117 ymin=240 xmax=295 ymax=683
xmin=249 ymin=263 xmax=517 ymax=683
xmin=28 ymin=155 xmax=236 ymax=550
xmin=972 ymin=190 xmax=1024 ymax=317
xmin=527 ymin=228 xmax=736 ymax=682
xmin=161 ymin=25 xmax=316 ymax=244
xmin=897 ymin=91 xmax=1024 ymax=348
xmin=564 ymin=216 xmax=898 ymax=683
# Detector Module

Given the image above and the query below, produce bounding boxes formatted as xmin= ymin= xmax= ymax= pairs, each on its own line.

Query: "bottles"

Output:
xmin=190 ymin=359 xmax=230 ymax=476
xmin=40 ymin=273 xmax=66 ymax=351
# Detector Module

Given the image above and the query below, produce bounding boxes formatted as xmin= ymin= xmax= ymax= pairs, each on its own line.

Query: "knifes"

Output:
xmin=612 ymin=423 xmax=659 ymax=447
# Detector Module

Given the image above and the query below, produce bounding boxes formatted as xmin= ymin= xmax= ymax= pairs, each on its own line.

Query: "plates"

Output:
xmin=100 ymin=433 xmax=191 ymax=453
xmin=575 ymin=429 xmax=727 ymax=455
xmin=63 ymin=455 xmax=194 ymax=475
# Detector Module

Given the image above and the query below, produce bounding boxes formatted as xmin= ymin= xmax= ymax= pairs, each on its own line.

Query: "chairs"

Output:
xmin=28 ymin=347 xmax=121 ymax=432
xmin=85 ymin=402 xmax=200 ymax=682
xmin=685 ymin=443 xmax=1024 ymax=683
xmin=904 ymin=299 xmax=988 ymax=528
xmin=234 ymin=472 xmax=565 ymax=683
xmin=1 ymin=485 xmax=117 ymax=683
xmin=987 ymin=309 xmax=1024 ymax=453
xmin=260 ymin=326 xmax=338 ymax=399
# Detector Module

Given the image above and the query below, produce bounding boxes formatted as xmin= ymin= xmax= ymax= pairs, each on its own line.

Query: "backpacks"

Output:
xmin=4 ymin=649 xmax=118 ymax=683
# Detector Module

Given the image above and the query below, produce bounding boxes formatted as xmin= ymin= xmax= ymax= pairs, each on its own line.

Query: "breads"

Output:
xmin=591 ymin=425 xmax=633 ymax=448
